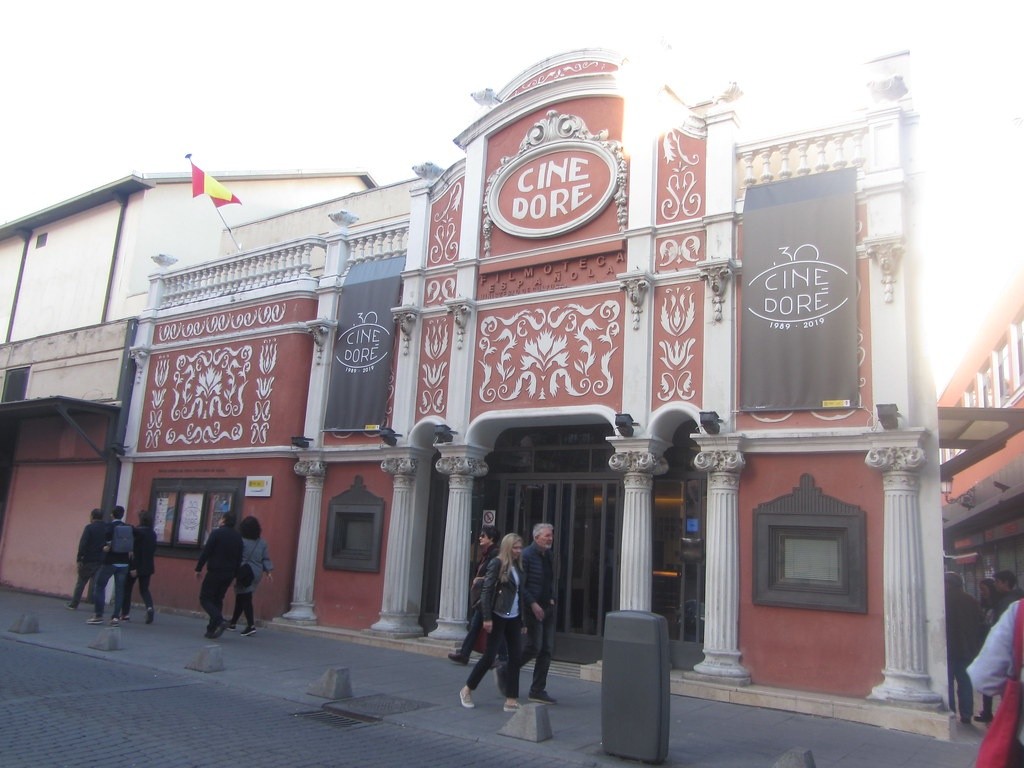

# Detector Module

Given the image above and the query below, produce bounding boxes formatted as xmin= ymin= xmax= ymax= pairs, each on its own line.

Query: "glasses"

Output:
xmin=479 ymin=533 xmax=485 ymax=538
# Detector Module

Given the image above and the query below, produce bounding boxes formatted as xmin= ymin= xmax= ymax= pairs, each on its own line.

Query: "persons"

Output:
xmin=195 ymin=511 xmax=242 ymax=638
xmin=460 ymin=533 xmax=525 ymax=711
xmin=65 ymin=508 xmax=106 ymax=609
xmin=86 ymin=506 xmax=137 ymax=627
xmin=944 ymin=570 xmax=1024 ymax=723
xmin=225 ymin=516 xmax=273 ymax=635
xmin=448 ymin=524 xmax=507 ymax=668
xmin=119 ymin=509 xmax=157 ymax=624
xmin=966 ymin=599 xmax=1024 ymax=768
xmin=494 ymin=524 xmax=557 ymax=703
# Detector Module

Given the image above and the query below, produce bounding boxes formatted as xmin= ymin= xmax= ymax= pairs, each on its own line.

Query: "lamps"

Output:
xmin=877 ymin=403 xmax=902 ymax=431
xmin=434 ymin=424 xmax=458 ymax=442
xmin=380 ymin=427 xmax=404 ymax=445
xmin=699 ymin=411 xmax=723 ymax=434
xmin=941 ymin=474 xmax=976 ymax=508
xmin=615 ymin=413 xmax=639 ymax=439
xmin=291 ymin=436 xmax=314 ymax=449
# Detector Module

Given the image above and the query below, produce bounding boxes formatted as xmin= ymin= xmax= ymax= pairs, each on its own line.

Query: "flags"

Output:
xmin=191 ymin=162 xmax=242 ymax=208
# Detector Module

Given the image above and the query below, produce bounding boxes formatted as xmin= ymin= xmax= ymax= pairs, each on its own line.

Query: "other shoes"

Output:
xmin=119 ymin=615 xmax=130 ymax=622
xmin=65 ymin=601 xmax=77 ymax=610
xmin=974 ymin=714 xmax=994 ymax=722
xmin=86 ymin=616 xmax=103 ymax=625
xmin=146 ymin=608 xmax=154 ymax=624
xmin=960 ymin=717 xmax=971 ymax=725
xmin=110 ymin=618 xmax=120 ymax=627
xmin=488 ymin=658 xmax=508 ymax=669
xmin=204 ymin=620 xmax=227 ymax=639
xmin=448 ymin=653 xmax=469 ymax=665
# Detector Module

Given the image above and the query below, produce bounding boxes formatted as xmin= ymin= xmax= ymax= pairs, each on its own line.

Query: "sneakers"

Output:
xmin=460 ymin=688 xmax=475 ymax=708
xmin=528 ymin=691 xmax=557 ymax=705
xmin=503 ymin=702 xmax=522 ymax=712
xmin=240 ymin=626 xmax=257 ymax=636
xmin=226 ymin=624 xmax=236 ymax=631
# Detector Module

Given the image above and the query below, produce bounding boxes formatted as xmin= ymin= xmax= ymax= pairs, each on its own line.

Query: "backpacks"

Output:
xmin=111 ymin=521 xmax=134 ymax=553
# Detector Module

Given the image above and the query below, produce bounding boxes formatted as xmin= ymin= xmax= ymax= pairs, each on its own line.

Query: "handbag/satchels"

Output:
xmin=237 ymin=563 xmax=254 ymax=587
xmin=469 ymin=556 xmax=504 ymax=613
xmin=975 ymin=599 xmax=1024 ymax=768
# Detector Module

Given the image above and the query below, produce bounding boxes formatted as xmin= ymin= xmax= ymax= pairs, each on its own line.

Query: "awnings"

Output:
xmin=0 ymin=396 xmax=122 ymax=459
